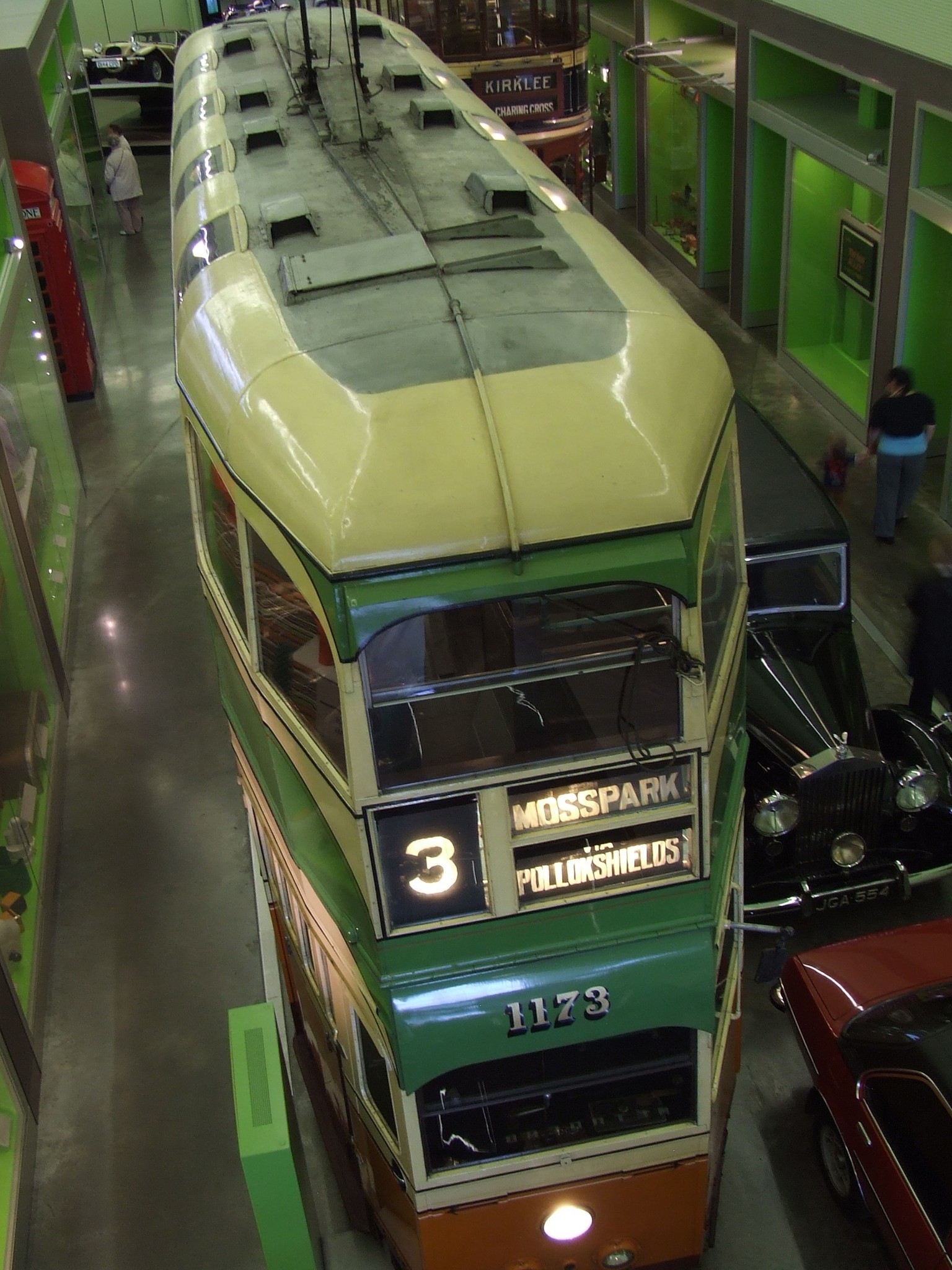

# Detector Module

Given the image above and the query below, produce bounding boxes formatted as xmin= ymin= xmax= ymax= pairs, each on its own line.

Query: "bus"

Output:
xmin=337 ymin=0 xmax=607 ymax=209
xmin=165 ymin=6 xmax=749 ymax=1270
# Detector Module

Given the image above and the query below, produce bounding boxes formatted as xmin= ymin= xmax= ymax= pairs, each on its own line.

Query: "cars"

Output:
xmin=695 ymin=392 xmax=952 ymax=976
xmin=770 ymin=911 xmax=952 ymax=1270
xmin=82 ymin=27 xmax=191 ymax=84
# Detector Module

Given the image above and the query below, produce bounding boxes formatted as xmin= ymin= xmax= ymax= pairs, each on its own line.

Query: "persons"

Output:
xmin=104 ymin=124 xmax=144 ymax=235
xmin=899 ymin=565 xmax=952 ymax=715
xmin=817 ymin=366 xmax=936 ymax=546
xmin=55 ymin=129 xmax=101 ymax=242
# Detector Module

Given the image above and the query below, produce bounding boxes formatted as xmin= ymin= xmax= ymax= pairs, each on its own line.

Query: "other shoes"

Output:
xmin=118 ymin=230 xmax=135 ymax=235
xmin=876 ymin=535 xmax=895 ymax=545
xmin=895 ymin=515 xmax=910 ymax=525
